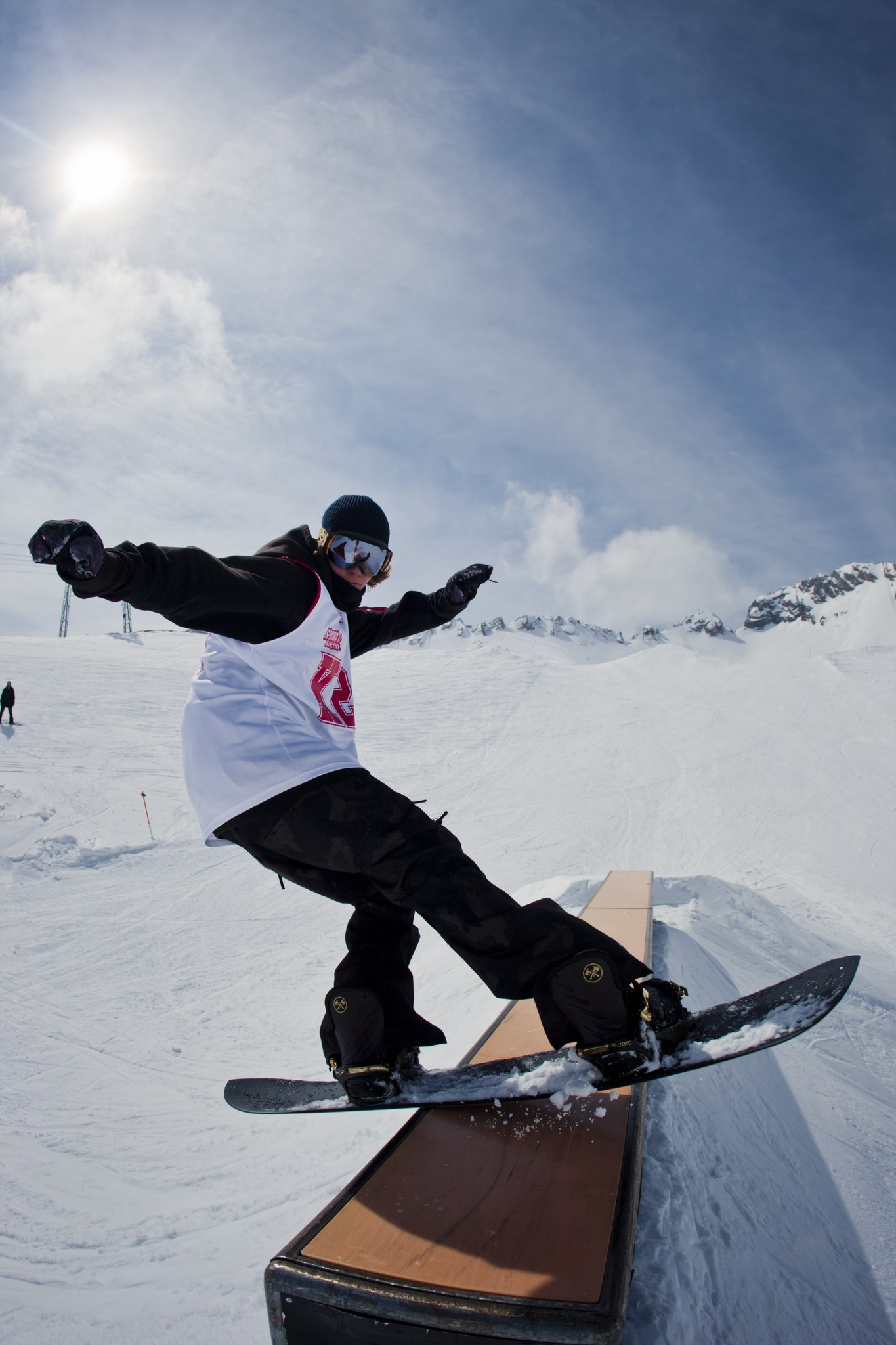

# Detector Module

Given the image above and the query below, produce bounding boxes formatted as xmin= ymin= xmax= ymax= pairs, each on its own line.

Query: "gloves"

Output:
xmin=446 ymin=564 xmax=493 ymax=605
xmin=28 ymin=519 xmax=105 ymax=580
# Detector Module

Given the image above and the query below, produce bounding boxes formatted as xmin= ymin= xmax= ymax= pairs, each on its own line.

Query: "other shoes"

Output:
xmin=531 ymin=947 xmax=691 ymax=1079
xmin=319 ymin=983 xmax=421 ymax=1104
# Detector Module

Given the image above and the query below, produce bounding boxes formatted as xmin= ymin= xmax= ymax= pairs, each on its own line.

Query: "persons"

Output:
xmin=0 ymin=681 xmax=15 ymax=724
xmin=26 ymin=494 xmax=695 ymax=1101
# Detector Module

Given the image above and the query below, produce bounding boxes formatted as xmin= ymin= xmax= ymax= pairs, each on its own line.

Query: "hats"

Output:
xmin=322 ymin=495 xmax=390 ymax=547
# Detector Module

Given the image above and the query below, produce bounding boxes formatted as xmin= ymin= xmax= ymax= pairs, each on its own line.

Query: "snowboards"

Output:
xmin=223 ymin=950 xmax=866 ymax=1117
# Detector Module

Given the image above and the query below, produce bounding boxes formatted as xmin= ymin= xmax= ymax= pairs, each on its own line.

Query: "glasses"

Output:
xmin=316 ymin=527 xmax=393 ymax=577
